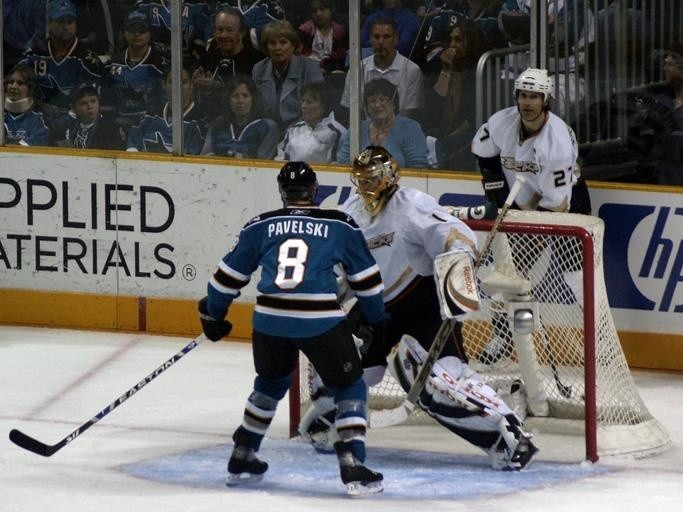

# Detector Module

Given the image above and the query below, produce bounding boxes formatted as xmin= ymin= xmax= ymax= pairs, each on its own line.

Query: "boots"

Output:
xmin=226 ymin=445 xmax=269 ymax=476
xmin=334 ymin=441 xmax=382 ymax=484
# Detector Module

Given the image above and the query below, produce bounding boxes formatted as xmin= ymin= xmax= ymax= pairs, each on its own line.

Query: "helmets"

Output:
xmin=278 ymin=162 xmax=317 ymax=203
xmin=350 ymin=147 xmax=403 ymax=217
xmin=514 ymin=67 xmax=555 ymax=109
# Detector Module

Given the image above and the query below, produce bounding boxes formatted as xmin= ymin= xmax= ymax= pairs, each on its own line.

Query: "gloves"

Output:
xmin=198 ymin=298 xmax=232 ymax=341
xmin=358 ymin=321 xmax=389 ymax=347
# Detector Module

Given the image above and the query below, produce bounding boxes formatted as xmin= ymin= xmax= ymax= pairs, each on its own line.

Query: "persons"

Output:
xmin=304 ymin=147 xmax=538 ymax=469
xmin=470 ymin=65 xmax=592 ymax=367
xmin=195 ymin=160 xmax=388 ymax=484
xmin=0 ymin=2 xmax=682 ymax=192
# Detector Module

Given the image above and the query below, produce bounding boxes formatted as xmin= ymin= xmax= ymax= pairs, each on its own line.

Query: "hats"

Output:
xmin=123 ymin=7 xmax=152 ymax=29
xmin=47 ymin=0 xmax=76 ymax=21
xmin=70 ymin=83 xmax=97 ymax=100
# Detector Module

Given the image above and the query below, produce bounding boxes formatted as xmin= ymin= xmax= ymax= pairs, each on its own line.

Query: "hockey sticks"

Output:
xmin=533 ymin=290 xmax=587 ymax=400
xmin=9 ymin=333 xmax=208 ymax=457
xmin=368 ymin=177 xmax=524 ymax=426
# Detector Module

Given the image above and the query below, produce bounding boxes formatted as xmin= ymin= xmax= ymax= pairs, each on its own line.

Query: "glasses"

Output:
xmin=126 ymin=27 xmax=146 ymax=34
